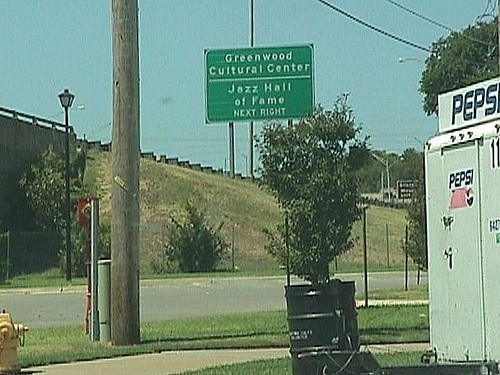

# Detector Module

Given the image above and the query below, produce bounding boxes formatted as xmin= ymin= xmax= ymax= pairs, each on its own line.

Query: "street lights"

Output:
xmin=58 ymin=88 xmax=76 ymax=284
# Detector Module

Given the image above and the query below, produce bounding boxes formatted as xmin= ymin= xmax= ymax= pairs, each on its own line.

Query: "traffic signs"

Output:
xmin=202 ymin=43 xmax=315 ymax=125
xmin=397 ymin=181 xmax=420 ymax=198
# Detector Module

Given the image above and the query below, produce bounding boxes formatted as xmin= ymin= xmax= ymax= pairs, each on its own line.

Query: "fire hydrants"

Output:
xmin=0 ymin=309 xmax=29 ymax=375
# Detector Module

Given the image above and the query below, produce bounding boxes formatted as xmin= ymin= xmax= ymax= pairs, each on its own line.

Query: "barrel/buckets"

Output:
xmin=285 ymin=283 xmax=358 ymax=374
